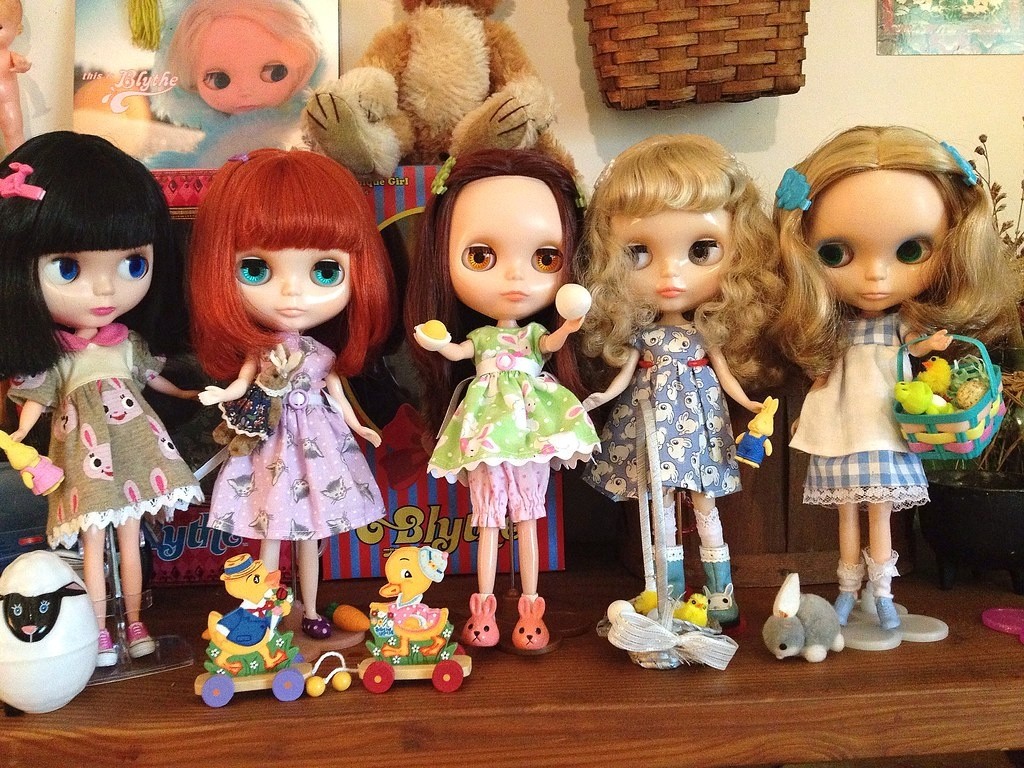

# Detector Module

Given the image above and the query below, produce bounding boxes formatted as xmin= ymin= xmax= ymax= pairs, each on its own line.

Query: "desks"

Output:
xmin=2 ymin=569 xmax=1024 ymax=768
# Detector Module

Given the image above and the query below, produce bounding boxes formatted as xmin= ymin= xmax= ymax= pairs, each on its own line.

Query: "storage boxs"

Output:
xmin=151 ymin=463 xmax=566 ymax=590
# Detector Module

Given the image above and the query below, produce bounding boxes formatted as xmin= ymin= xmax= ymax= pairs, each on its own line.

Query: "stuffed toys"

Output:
xmin=301 ymin=0 xmax=579 ymax=178
xmin=734 ymin=396 xmax=779 ymax=468
xmin=634 ymin=591 xmax=657 ymax=614
xmin=763 ymin=573 xmax=844 ymax=663
xmin=896 ymin=382 xmax=952 ymax=415
xmin=0 ymin=429 xmax=66 ymax=496
xmin=674 ymin=593 xmax=707 ymax=627
xmin=918 ymin=356 xmax=953 ymax=402
xmin=212 ymin=345 xmax=305 ymax=456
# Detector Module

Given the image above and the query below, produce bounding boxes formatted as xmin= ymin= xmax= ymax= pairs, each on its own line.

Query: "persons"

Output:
xmin=0 ymin=132 xmax=203 ymax=666
xmin=772 ymin=126 xmax=1024 ymax=631
xmin=0 ymin=0 xmax=33 ymax=155
xmin=188 ymin=149 xmax=390 ymax=640
xmin=578 ymin=135 xmax=786 ymax=622
xmin=404 ymin=147 xmax=601 ymax=651
